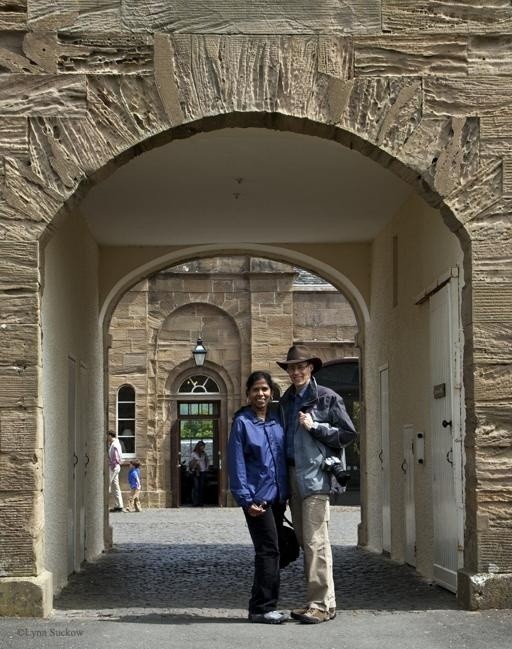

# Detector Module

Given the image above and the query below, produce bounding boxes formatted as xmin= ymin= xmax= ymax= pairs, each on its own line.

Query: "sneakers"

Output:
xmin=298 ymin=606 xmax=337 ymax=624
xmin=276 ymin=609 xmax=293 ymax=621
xmin=109 ymin=505 xmax=142 ymax=513
xmin=248 ymin=612 xmax=289 ymax=624
xmin=291 ymin=606 xmax=309 ymax=621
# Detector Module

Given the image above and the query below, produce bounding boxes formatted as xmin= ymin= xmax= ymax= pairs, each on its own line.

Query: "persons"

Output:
xmin=189 ymin=441 xmax=210 ymax=506
xmin=225 ymin=370 xmax=291 ymax=623
xmin=122 ymin=460 xmax=143 ymax=513
xmin=232 ymin=344 xmax=358 ymax=626
xmin=107 ymin=428 xmax=125 ymax=512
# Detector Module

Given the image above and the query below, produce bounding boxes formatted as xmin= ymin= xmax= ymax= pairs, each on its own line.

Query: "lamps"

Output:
xmin=192 ymin=337 xmax=207 ymax=367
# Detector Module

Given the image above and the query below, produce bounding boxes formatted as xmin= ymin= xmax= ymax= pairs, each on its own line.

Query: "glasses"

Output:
xmin=286 ymin=362 xmax=312 ymax=373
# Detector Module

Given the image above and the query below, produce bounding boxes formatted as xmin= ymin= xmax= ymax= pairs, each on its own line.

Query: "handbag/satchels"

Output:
xmin=277 ymin=524 xmax=300 ymax=569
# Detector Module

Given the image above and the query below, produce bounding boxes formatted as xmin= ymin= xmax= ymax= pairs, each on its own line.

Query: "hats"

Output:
xmin=275 ymin=345 xmax=323 ymax=375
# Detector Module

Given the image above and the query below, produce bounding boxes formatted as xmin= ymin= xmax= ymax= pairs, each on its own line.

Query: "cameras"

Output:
xmin=321 ymin=456 xmax=351 ymax=488
